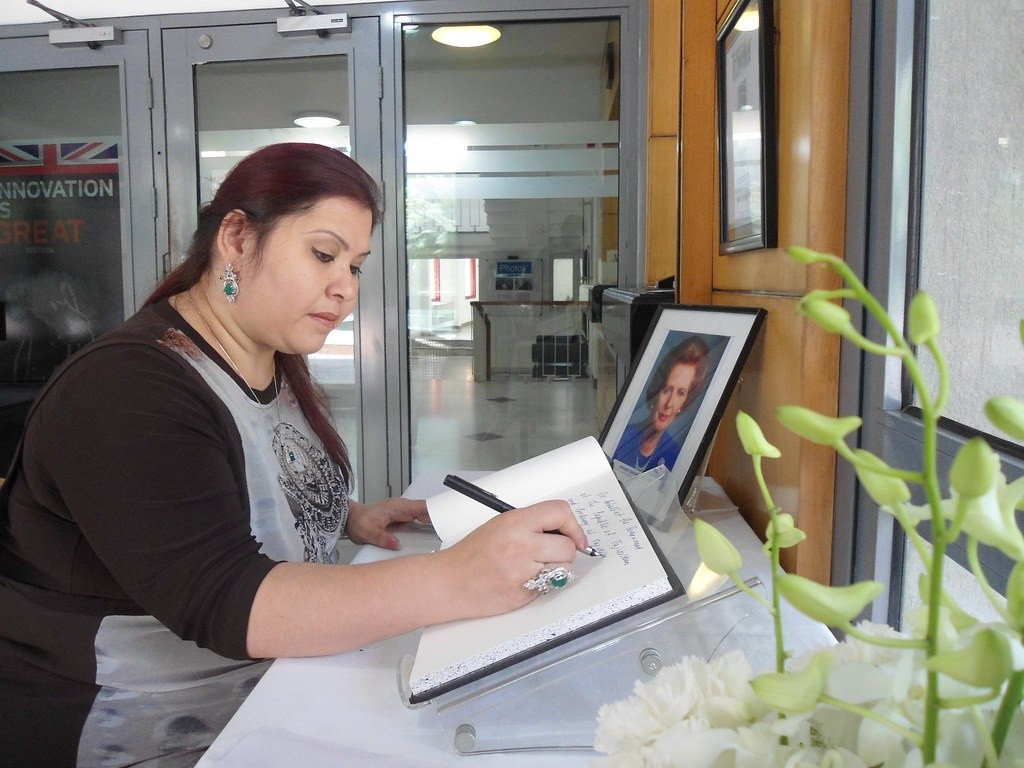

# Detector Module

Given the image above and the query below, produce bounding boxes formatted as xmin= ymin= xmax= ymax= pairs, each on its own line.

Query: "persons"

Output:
xmin=611 ymin=334 xmax=710 ymax=492
xmin=0 ymin=142 xmax=589 ymax=768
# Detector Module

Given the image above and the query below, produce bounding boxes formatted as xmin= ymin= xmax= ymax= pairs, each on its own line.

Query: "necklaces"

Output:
xmin=189 ymin=293 xmax=296 ymax=463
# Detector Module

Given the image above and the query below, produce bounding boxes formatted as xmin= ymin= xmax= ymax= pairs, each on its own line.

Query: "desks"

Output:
xmin=195 ymin=468 xmax=855 ymax=766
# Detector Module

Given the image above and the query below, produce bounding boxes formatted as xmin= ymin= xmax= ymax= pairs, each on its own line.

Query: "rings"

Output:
xmin=522 ymin=561 xmax=580 ymax=596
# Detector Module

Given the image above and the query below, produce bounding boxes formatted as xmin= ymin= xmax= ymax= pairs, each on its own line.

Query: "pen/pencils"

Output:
xmin=443 ymin=474 xmax=605 ymax=558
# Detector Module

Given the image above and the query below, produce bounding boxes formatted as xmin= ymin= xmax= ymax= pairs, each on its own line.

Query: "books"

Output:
xmin=409 ymin=435 xmax=687 ymax=705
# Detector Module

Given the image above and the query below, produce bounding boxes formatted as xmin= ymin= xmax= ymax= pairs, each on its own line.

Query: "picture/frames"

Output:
xmin=716 ymin=0 xmax=779 ymax=254
xmin=598 ymin=304 xmax=770 ymax=536
